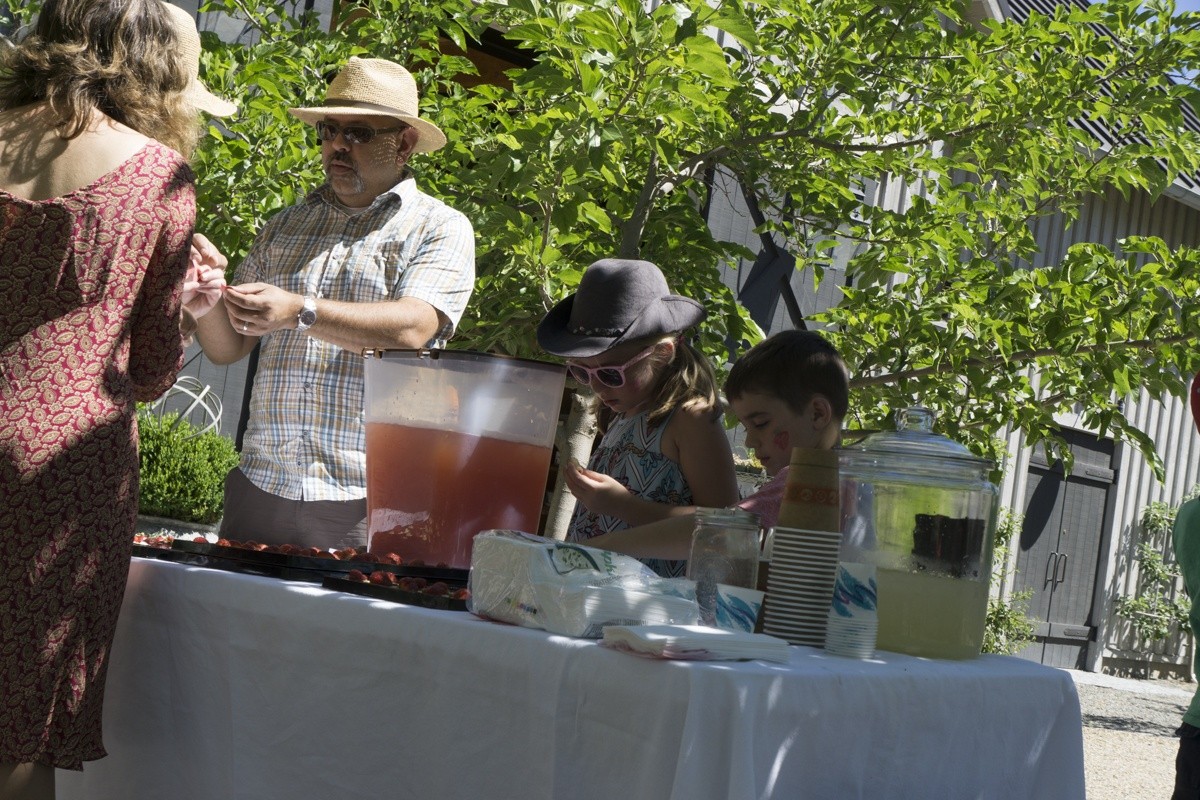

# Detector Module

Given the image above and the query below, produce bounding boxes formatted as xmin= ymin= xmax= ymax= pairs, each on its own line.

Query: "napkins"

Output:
xmin=603 ymin=618 xmax=788 ymax=665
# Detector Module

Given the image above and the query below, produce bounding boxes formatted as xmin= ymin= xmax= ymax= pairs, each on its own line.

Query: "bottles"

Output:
xmin=686 ymin=507 xmax=762 ymax=626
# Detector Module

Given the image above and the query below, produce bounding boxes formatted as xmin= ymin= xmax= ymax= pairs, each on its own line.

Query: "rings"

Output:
xmin=243 ymin=321 xmax=248 ymax=331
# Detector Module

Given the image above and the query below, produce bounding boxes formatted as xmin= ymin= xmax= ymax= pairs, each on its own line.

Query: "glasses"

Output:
xmin=314 ymin=120 xmax=408 ymax=145
xmin=565 ymin=339 xmax=677 ymax=387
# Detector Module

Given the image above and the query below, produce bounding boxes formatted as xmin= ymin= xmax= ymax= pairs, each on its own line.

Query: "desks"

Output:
xmin=55 ymin=557 xmax=1085 ymax=798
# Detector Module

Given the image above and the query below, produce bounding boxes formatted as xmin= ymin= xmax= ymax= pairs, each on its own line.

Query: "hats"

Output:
xmin=160 ymin=1 xmax=238 ymax=116
xmin=536 ymin=258 xmax=708 ymax=358
xmin=287 ymin=55 xmax=447 ymax=153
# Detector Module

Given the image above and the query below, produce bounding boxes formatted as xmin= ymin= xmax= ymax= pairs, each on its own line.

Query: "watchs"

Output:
xmin=294 ymin=296 xmax=315 ymax=331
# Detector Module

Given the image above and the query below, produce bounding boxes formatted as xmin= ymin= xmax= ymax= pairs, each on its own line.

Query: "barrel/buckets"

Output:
xmin=361 ymin=347 xmax=569 ymax=568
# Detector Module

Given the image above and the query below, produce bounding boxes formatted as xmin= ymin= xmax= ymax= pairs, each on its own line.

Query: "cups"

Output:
xmin=714 ymin=583 xmax=766 ymax=634
xmin=823 ymin=561 xmax=879 ymax=658
xmin=762 ymin=447 xmax=845 ymax=649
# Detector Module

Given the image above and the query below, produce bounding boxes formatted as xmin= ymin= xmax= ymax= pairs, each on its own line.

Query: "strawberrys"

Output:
xmin=134 ymin=532 xmax=471 ymax=599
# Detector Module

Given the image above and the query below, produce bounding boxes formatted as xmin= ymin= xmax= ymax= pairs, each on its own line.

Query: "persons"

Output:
xmin=1169 ymin=371 xmax=1200 ymax=800
xmin=536 ymin=258 xmax=879 ymax=634
xmin=183 ymin=56 xmax=472 ymax=556
xmin=0 ymin=1 xmax=240 ymax=800
